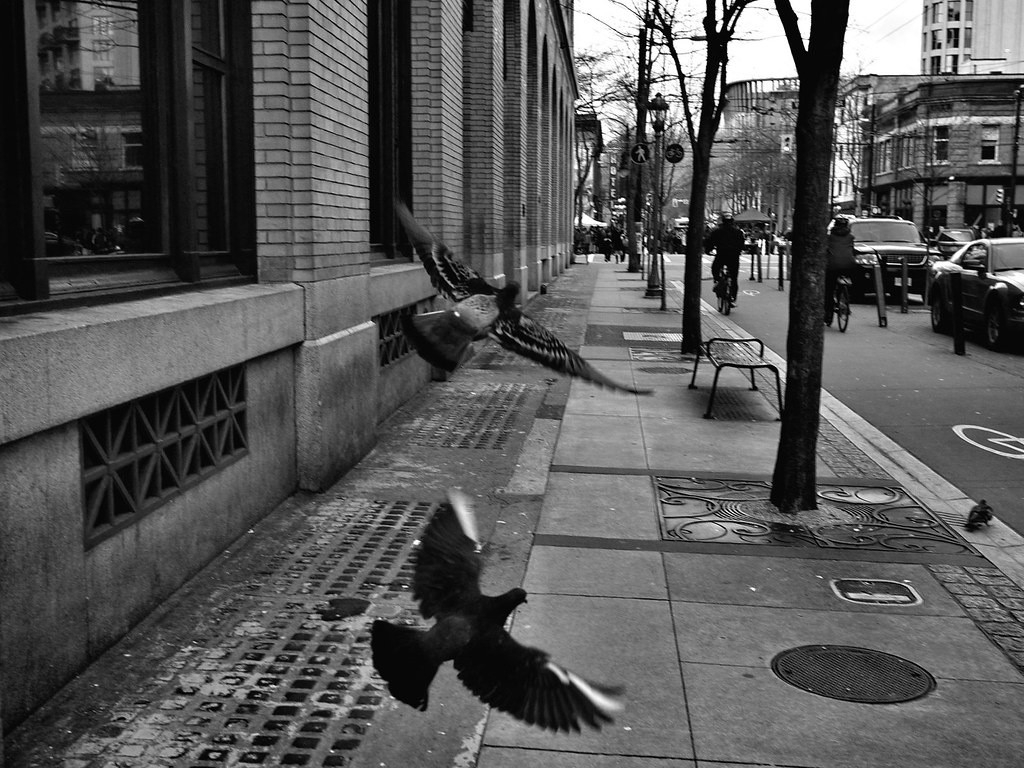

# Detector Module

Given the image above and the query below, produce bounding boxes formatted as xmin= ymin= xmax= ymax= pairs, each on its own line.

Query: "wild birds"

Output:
xmin=965 ymin=499 xmax=995 ymax=531
xmin=389 ymin=194 xmax=657 ymax=398
xmin=370 ymin=485 xmax=629 ymax=735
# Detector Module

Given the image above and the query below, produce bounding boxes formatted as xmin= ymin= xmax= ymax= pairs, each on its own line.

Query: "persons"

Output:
xmin=703 ymin=216 xmax=745 ymax=309
xmin=823 ymin=219 xmax=855 ymax=324
xmin=572 ymin=214 xmax=666 ymax=266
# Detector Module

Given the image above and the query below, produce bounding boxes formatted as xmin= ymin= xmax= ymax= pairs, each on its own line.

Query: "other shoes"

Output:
xmin=604 ymin=258 xmax=609 ymax=262
xmin=713 ymin=281 xmax=720 ymax=292
xmin=824 ymin=317 xmax=833 ymax=327
xmin=731 ymin=301 xmax=738 ymax=307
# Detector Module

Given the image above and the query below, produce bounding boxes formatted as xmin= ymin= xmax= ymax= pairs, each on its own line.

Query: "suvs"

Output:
xmin=827 ymin=216 xmax=945 ymax=305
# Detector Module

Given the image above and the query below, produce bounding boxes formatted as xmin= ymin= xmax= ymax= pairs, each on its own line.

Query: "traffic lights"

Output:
xmin=996 ymin=188 xmax=1005 ymax=205
xmin=872 ymin=206 xmax=879 ymax=214
xmin=780 ymin=133 xmax=794 ymax=154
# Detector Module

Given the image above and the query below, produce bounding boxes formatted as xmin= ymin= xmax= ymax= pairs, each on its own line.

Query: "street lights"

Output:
xmin=645 ymin=92 xmax=670 ymax=298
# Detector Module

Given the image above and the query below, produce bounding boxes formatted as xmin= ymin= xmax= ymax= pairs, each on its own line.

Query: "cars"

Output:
xmin=926 ymin=238 xmax=1024 ymax=352
xmin=926 ymin=228 xmax=976 ymax=263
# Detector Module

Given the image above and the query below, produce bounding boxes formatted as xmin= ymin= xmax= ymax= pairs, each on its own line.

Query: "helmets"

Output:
xmin=833 ymin=214 xmax=848 ymax=220
xmin=717 ymin=207 xmax=733 ymax=221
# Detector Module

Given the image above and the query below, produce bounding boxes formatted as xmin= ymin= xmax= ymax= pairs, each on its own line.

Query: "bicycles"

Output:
xmin=707 ymin=251 xmax=734 ymax=316
xmin=834 ymin=272 xmax=852 ymax=333
